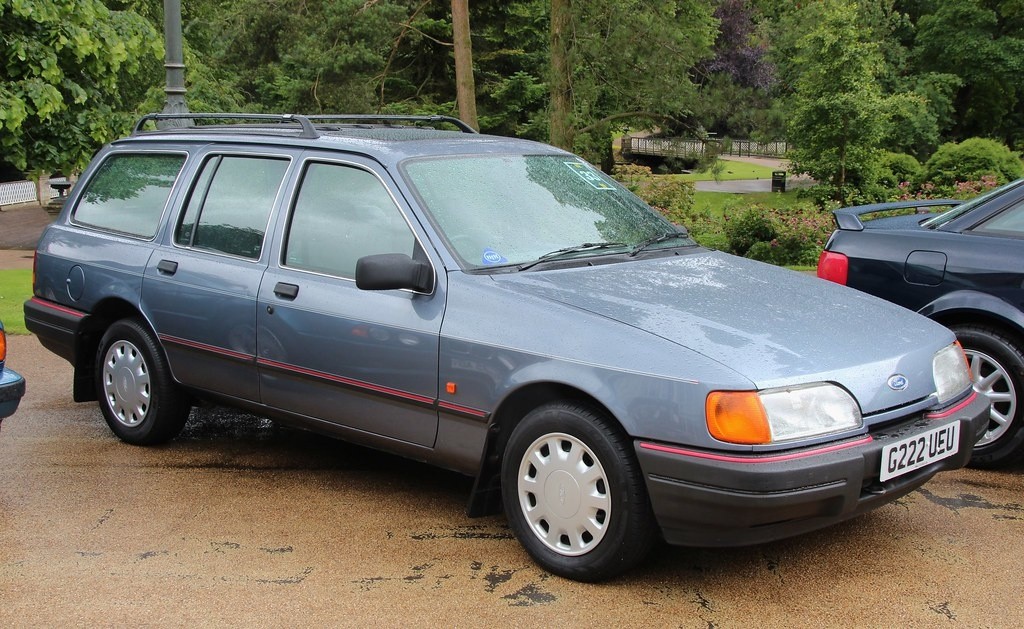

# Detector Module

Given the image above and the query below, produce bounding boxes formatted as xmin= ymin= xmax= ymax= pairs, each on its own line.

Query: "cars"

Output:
xmin=817 ymin=175 xmax=1024 ymax=471
xmin=22 ymin=109 xmax=993 ymax=586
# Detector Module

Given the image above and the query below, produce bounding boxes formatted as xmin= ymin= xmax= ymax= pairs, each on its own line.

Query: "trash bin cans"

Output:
xmin=772 ymin=170 xmax=786 ymax=192
